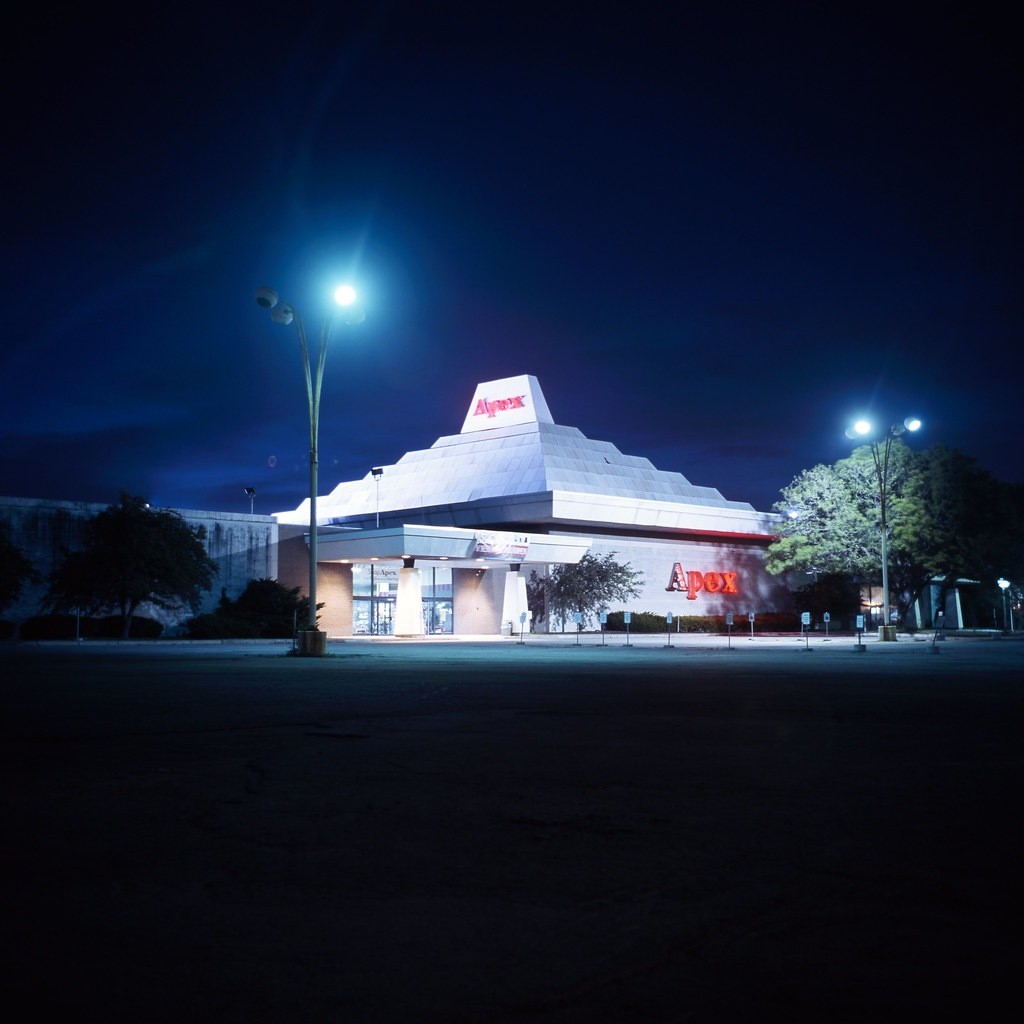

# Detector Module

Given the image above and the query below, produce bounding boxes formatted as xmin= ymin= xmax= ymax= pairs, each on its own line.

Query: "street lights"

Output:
xmin=999 ymin=580 xmax=1014 ymax=631
xmin=845 ymin=416 xmax=923 ymax=640
xmin=255 ymin=280 xmax=357 ymax=647
